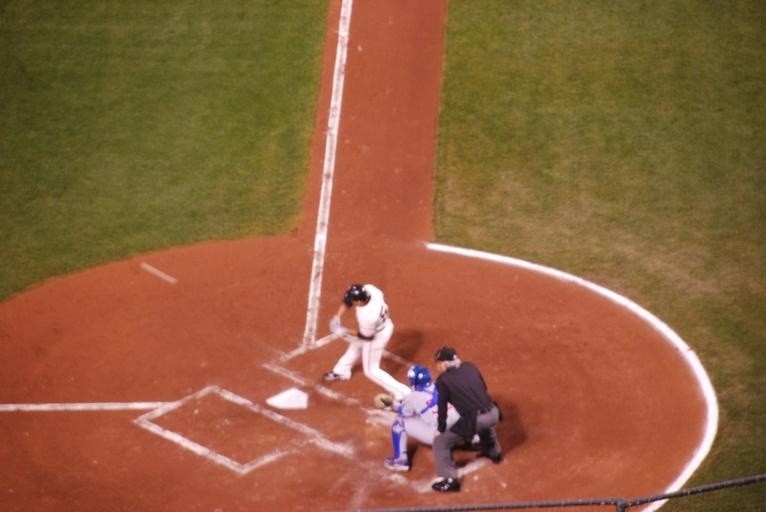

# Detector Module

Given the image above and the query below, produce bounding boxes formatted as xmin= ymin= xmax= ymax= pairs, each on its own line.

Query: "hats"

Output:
xmin=434 ymin=346 xmax=457 ymax=361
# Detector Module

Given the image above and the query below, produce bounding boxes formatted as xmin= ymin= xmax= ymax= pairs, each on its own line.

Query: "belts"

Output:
xmin=481 ymin=406 xmax=493 ymax=413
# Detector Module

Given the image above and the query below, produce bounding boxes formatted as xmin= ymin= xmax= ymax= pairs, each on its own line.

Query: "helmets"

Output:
xmin=344 ymin=283 xmax=368 ymax=307
xmin=408 ymin=365 xmax=431 ymax=389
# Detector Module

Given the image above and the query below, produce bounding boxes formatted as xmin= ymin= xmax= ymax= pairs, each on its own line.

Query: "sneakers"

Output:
xmin=384 ymin=458 xmax=409 ymax=472
xmin=324 ymin=371 xmax=349 ymax=381
xmin=432 ymin=478 xmax=460 ymax=491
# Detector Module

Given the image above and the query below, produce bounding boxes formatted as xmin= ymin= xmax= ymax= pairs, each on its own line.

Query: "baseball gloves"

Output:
xmin=375 ymin=395 xmax=393 ymax=410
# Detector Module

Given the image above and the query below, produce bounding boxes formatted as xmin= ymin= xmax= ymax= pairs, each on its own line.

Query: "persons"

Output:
xmin=429 ymin=344 xmax=504 ymax=490
xmin=325 ymin=282 xmax=411 ymax=401
xmin=380 ymin=362 xmax=465 ymax=473
